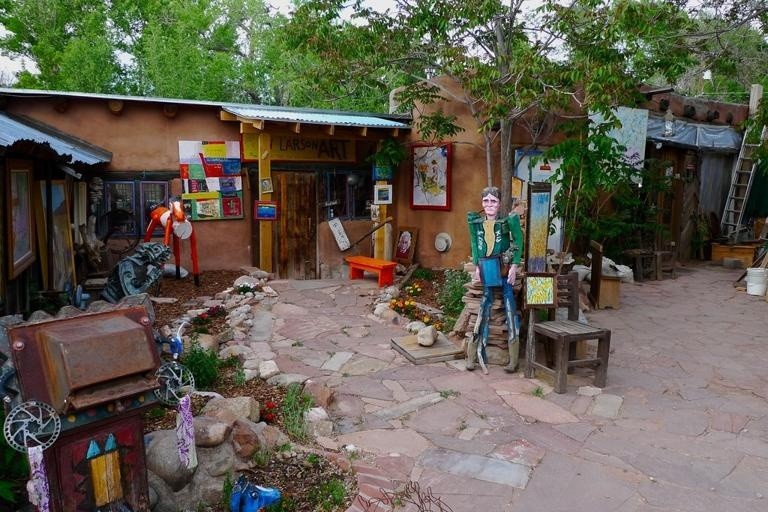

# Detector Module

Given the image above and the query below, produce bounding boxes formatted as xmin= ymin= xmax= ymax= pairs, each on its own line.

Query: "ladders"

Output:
xmin=717 ymin=124 xmax=767 ymax=245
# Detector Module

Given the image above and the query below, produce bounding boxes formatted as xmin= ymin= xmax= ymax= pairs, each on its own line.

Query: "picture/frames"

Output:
xmin=254 ymin=200 xmax=277 ymax=220
xmin=523 ymin=272 xmax=558 ymax=308
xmin=374 ymin=185 xmax=392 ymax=204
xmin=410 ymin=142 xmax=452 ymax=211
xmin=394 ymin=226 xmax=418 ymax=263
xmin=5 ymin=159 xmax=36 ymax=280
xmin=259 ymin=177 xmax=273 ymax=194
xmin=35 ymin=181 xmax=77 ymax=291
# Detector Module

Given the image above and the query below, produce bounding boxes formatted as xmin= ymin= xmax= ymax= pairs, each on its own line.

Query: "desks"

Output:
xmin=624 ymin=254 xmax=656 ymax=281
xmin=712 ymin=243 xmax=754 ymax=268
xmin=655 ymin=251 xmax=677 ymax=281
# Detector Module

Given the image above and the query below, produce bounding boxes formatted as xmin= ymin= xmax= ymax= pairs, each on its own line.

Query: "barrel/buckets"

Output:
xmin=746 ymin=267 xmax=768 ymax=296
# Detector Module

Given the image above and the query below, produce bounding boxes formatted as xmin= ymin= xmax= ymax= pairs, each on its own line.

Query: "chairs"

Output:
xmin=525 ymin=271 xmax=611 ymax=393
xmin=591 ymin=240 xmax=625 ymax=309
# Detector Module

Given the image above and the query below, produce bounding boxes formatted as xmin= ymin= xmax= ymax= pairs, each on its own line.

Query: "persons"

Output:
xmin=466 ymin=187 xmax=523 ymax=373
xmin=398 ymin=230 xmax=411 ymax=258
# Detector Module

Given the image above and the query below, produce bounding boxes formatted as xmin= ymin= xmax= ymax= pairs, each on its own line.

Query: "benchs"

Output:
xmin=345 ymin=256 xmax=396 ymax=287
